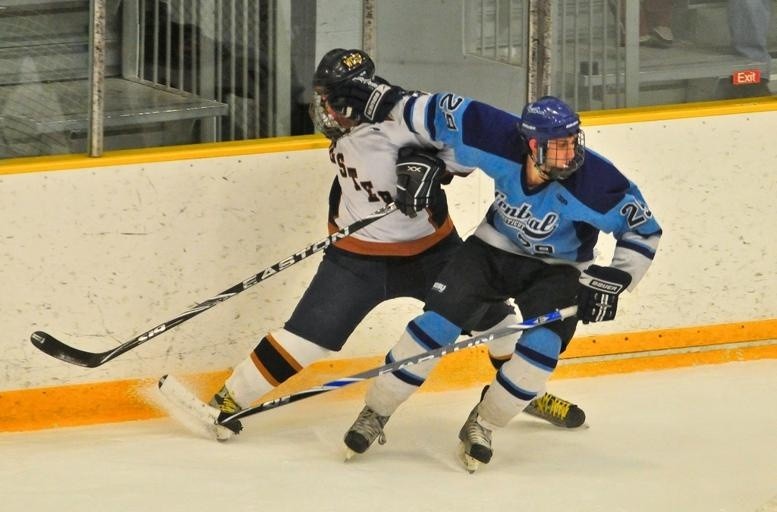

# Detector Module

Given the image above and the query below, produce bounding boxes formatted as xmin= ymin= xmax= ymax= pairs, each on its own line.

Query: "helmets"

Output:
xmin=521 ymin=96 xmax=582 ymax=168
xmin=309 ymin=49 xmax=375 ymax=139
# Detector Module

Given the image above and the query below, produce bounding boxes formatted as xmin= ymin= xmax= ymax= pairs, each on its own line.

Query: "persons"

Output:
xmin=323 ymin=68 xmax=661 ymax=466
xmin=110 ymin=1 xmax=316 ymax=140
xmin=204 ymin=47 xmax=586 ymax=438
xmin=607 ymin=1 xmax=677 ymax=48
xmin=672 ymin=1 xmax=775 ymax=103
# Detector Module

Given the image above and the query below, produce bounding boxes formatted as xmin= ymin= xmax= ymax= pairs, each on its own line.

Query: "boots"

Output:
xmin=522 ymin=393 xmax=586 ymax=427
xmin=345 ymin=406 xmax=391 ymax=454
xmin=213 ymin=392 xmax=241 ymax=441
xmin=461 ymin=385 xmax=498 ymax=463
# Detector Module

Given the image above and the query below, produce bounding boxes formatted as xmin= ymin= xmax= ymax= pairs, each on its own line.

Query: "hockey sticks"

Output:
xmin=158 ymin=306 xmax=582 ymax=426
xmin=31 ymin=200 xmax=402 ymax=367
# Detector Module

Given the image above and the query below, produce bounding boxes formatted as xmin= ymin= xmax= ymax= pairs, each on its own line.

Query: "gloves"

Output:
xmin=327 ymin=75 xmax=402 ymax=124
xmin=396 ymin=147 xmax=445 ymax=217
xmin=576 ymin=265 xmax=632 ymax=322
